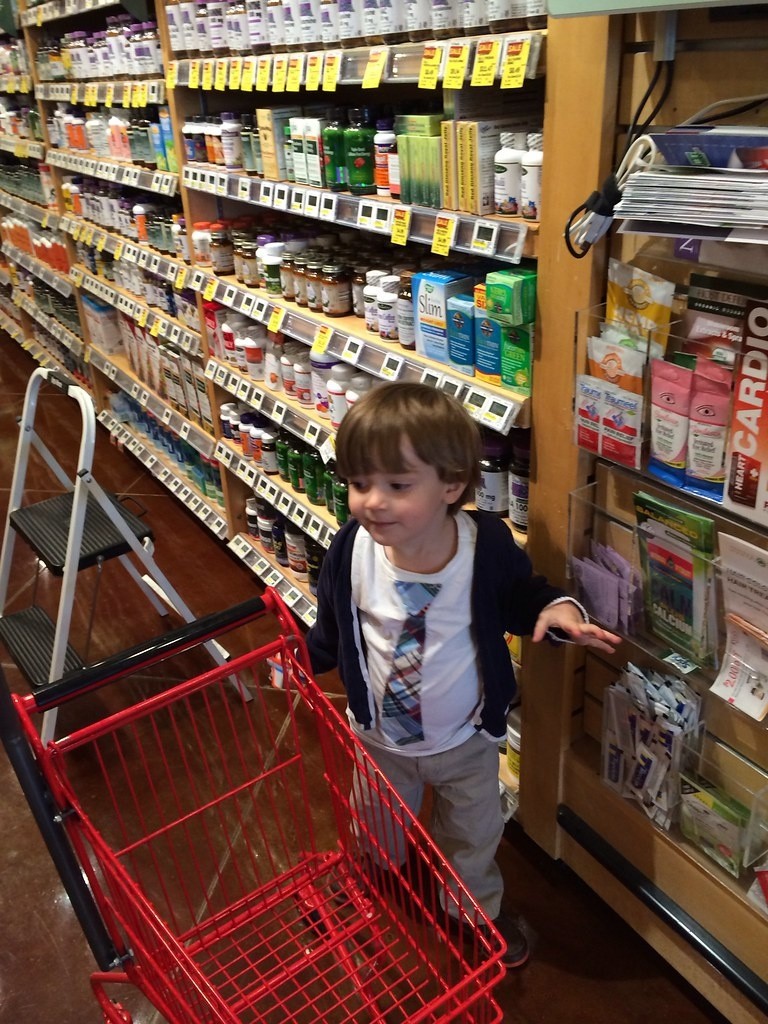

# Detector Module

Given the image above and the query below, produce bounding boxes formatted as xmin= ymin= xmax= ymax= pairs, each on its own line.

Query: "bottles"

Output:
xmin=518 ymin=132 xmax=544 ymax=222
xmin=494 ymin=133 xmax=521 ymax=217
xmin=192 ymin=217 xmax=429 ymax=595
xmin=508 ymin=444 xmax=530 ymax=533
xmin=387 ymin=124 xmax=402 ymax=200
xmin=283 ymin=126 xmax=294 ymax=182
xmin=10 ymin=174 xmax=190 ymax=328
xmin=344 ymin=109 xmax=375 ymax=194
xmin=472 ymin=440 xmax=509 ymax=517
xmin=181 ymin=113 xmax=263 ymax=175
xmin=37 ymin=0 xmax=547 ymax=82
xmin=374 ymin=121 xmax=397 ymax=197
xmin=54 ymin=105 xmax=158 ymax=170
xmin=322 ymin=109 xmax=345 ymax=193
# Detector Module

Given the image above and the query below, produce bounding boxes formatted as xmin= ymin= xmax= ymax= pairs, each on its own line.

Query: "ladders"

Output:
xmin=0 ymin=368 xmax=257 ymax=764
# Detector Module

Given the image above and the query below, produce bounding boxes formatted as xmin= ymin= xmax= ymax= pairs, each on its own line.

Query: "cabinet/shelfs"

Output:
xmin=0 ymin=0 xmax=768 ymax=1024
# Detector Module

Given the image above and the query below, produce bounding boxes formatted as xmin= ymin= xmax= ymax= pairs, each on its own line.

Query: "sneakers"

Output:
xmin=325 ymin=849 xmax=417 ymax=904
xmin=450 ymin=912 xmax=530 ymax=967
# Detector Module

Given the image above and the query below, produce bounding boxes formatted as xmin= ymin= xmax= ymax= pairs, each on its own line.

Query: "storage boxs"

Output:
xmin=288 ymin=115 xmax=310 ymax=186
xmin=411 ymin=266 xmax=474 ymax=364
xmin=392 ymin=83 xmax=543 ymax=218
xmin=255 ymin=105 xmax=303 ymax=183
xmin=473 ymin=282 xmax=501 ymax=388
xmin=445 ymin=290 xmax=474 ymax=365
xmin=79 ymin=292 xmax=215 ymax=435
xmin=303 ymin=117 xmax=327 ymax=190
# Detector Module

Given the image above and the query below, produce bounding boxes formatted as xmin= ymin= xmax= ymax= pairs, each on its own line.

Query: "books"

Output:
xmin=612 ymin=124 xmax=768 ymax=244
xmin=632 ymin=491 xmax=768 ymax=722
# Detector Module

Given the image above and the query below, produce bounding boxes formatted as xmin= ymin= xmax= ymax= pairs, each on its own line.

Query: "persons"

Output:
xmin=267 ymin=380 xmax=621 ymax=968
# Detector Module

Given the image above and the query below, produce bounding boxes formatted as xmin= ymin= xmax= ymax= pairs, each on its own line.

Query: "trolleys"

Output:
xmin=0 ymin=585 xmax=507 ymax=1024
